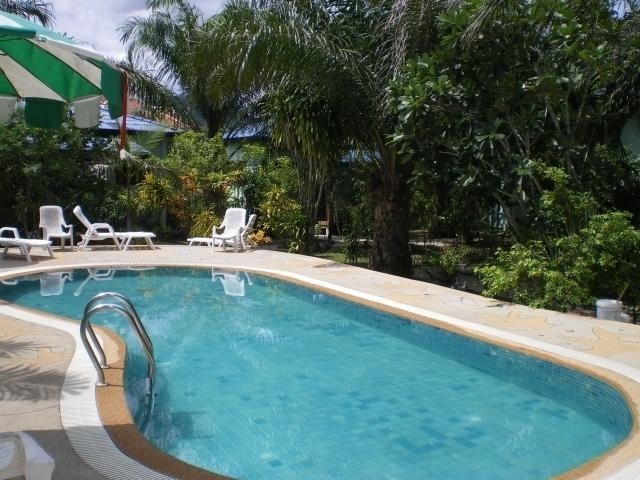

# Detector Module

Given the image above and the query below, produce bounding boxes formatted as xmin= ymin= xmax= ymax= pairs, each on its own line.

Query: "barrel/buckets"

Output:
xmin=594 ymin=299 xmax=623 ymax=321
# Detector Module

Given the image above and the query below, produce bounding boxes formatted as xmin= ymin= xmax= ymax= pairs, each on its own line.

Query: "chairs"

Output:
xmin=40 ymin=270 xmax=73 ymax=298
xmin=38 ymin=205 xmax=73 ymax=251
xmin=73 ymin=205 xmax=157 ymax=253
xmin=0 ymin=226 xmax=56 ymax=263
xmin=186 ymin=213 xmax=258 ymax=250
xmin=212 ymin=268 xmax=253 ymax=297
xmin=74 ymin=266 xmax=155 ymax=297
xmin=1 ymin=274 xmax=40 ymax=286
xmin=212 ymin=207 xmax=248 ymax=255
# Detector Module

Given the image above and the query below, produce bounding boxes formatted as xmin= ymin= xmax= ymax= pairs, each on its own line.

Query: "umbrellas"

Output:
xmin=0 ymin=9 xmax=129 ymax=133
